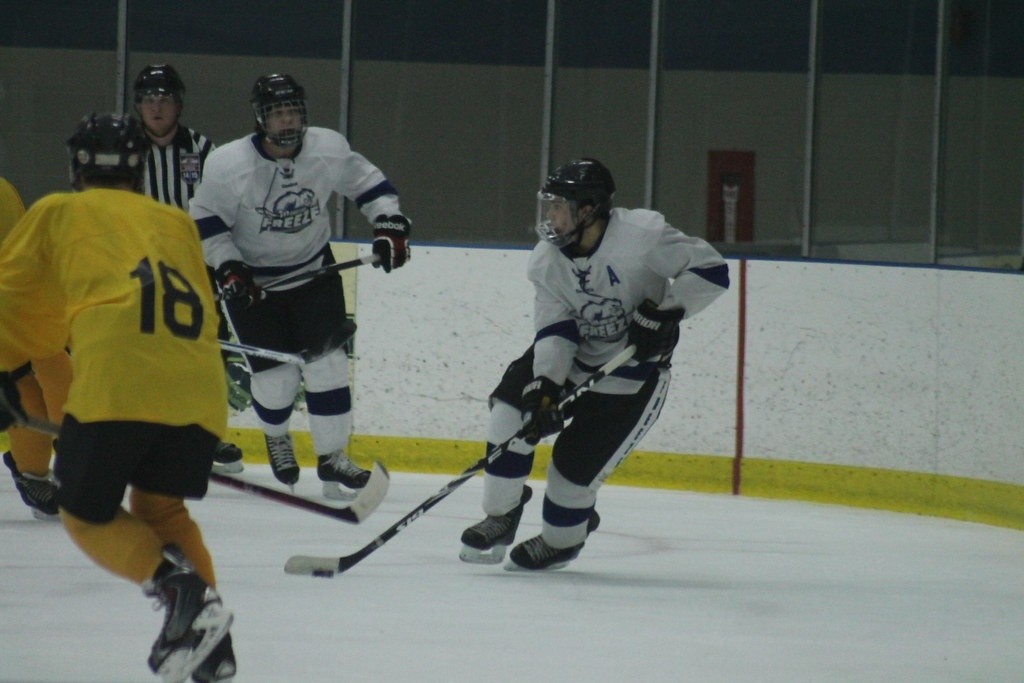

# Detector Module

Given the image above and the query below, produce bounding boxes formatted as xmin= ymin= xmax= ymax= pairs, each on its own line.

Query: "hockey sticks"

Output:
xmin=213 ymin=252 xmax=382 ymax=302
xmin=12 ymin=413 xmax=391 ymax=524
xmin=283 ymin=343 xmax=638 ymax=578
xmin=218 ymin=320 xmax=357 ymax=366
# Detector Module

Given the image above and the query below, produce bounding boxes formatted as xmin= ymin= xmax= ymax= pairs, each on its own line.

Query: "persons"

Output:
xmin=1 ymin=112 xmax=237 ymax=682
xmin=127 ymin=64 xmax=243 ymax=473
xmin=0 ymin=176 xmax=69 ymax=517
xmin=461 ymin=155 xmax=730 ymax=571
xmin=189 ymin=72 xmax=411 ymax=497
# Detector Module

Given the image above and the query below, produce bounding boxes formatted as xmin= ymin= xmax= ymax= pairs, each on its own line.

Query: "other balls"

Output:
xmin=312 ymin=569 xmax=334 ymax=577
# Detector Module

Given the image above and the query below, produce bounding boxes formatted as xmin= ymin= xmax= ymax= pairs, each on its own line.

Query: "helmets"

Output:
xmin=251 ymin=73 xmax=309 ymax=148
xmin=133 ymin=65 xmax=185 ymax=109
xmin=541 ymin=157 xmax=615 ymax=248
xmin=65 ymin=112 xmax=150 ymax=182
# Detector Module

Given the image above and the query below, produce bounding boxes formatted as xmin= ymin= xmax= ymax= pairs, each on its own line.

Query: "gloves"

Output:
xmin=627 ymin=296 xmax=686 ymax=359
xmin=521 ymin=375 xmax=567 ymax=446
xmin=372 ymin=215 xmax=412 ymax=274
xmin=216 ymin=261 xmax=267 ymax=307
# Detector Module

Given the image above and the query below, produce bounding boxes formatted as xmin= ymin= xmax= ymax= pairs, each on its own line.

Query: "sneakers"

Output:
xmin=3 ymin=451 xmax=65 ymax=515
xmin=318 ymin=449 xmax=371 ymax=488
xmin=510 ymin=508 xmax=600 ymax=570
xmin=146 ymin=542 xmax=238 ymax=683
xmin=265 ymin=432 xmax=300 ymax=486
xmin=460 ymin=484 xmax=532 ymax=548
xmin=213 ymin=442 xmax=243 ymax=463
